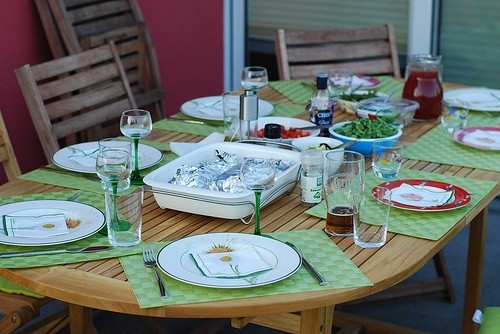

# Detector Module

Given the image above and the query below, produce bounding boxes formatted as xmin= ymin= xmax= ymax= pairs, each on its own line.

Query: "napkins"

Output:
xmin=470 ymin=92 xmax=500 ymax=106
xmin=189 ymin=246 xmax=272 ymax=278
xmin=170 ymin=132 xmax=224 ymax=159
xmin=386 ymin=182 xmax=454 ymax=208
xmin=2 ymin=213 xmax=69 ymax=237
xmin=69 ymin=148 xmax=141 ymax=169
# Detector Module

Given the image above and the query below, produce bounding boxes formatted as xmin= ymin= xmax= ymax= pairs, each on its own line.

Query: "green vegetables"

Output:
xmin=333 ymin=117 xmax=398 ymax=139
xmin=339 ymin=89 xmax=378 ymax=101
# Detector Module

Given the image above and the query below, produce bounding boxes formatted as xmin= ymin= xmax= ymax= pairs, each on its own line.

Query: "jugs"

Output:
xmin=403 ymin=52 xmax=446 ymax=121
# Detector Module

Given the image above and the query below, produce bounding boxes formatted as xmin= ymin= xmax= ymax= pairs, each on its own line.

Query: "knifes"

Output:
xmin=284 ymin=241 xmax=327 ymax=287
xmin=165 ymin=116 xmax=224 ymax=127
xmin=0 ymin=245 xmax=116 ymax=259
xmin=39 ymin=166 xmax=101 ymax=182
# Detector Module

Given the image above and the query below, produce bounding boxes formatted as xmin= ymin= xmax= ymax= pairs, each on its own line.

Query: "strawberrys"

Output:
xmin=246 ymin=125 xmax=310 ymax=139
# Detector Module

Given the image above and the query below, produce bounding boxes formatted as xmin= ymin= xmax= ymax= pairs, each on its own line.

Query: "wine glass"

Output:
xmin=95 ymin=150 xmax=132 ymax=235
xmin=119 ymin=109 xmax=153 ymax=185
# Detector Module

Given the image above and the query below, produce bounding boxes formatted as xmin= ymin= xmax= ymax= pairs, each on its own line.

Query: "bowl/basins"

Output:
xmin=142 ymin=90 xmax=420 ymax=219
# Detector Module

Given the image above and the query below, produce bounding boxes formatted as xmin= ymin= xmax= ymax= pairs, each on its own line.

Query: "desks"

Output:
xmin=0 ymin=76 xmax=500 ymax=334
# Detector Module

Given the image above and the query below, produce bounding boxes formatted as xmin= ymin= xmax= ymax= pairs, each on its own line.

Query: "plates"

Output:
xmin=442 ymin=87 xmax=500 ymax=112
xmin=0 ymin=200 xmax=105 ymax=246
xmin=453 ymin=126 xmax=500 ymax=152
xmin=328 ymin=74 xmax=382 ymax=90
xmin=372 ymin=179 xmax=471 ymax=212
xmin=181 ymin=96 xmax=274 ymax=121
xmin=155 ymin=232 xmax=302 ymax=289
xmin=49 ymin=141 xmax=164 ymax=172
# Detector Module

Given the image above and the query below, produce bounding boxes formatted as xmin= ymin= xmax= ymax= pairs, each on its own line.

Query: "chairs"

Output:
xmin=0 ymin=0 xmax=462 ymax=307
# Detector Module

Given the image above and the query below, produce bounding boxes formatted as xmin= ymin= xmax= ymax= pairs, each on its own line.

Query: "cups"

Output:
xmin=439 ymin=99 xmax=470 ymax=134
xmin=105 ymin=185 xmax=144 ymax=247
xmin=323 ymin=150 xmax=366 ymax=237
xmin=352 ymin=184 xmax=390 ymax=247
xmin=99 ymin=138 xmax=131 ymax=191
xmin=223 ymin=91 xmax=240 ymax=142
xmin=371 ymin=140 xmax=406 ymax=180
xmin=240 ymin=67 xmax=269 ymax=89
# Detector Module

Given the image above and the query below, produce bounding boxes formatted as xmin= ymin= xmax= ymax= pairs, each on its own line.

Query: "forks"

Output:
xmin=67 ymin=192 xmax=80 ymax=201
xmin=142 ymin=248 xmax=168 ymax=300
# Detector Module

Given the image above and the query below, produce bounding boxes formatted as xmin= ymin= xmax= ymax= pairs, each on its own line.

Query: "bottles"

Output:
xmin=308 ymin=72 xmax=333 ymax=137
xmin=241 ymin=91 xmax=259 ymax=141
xmin=299 ymin=149 xmax=326 ymax=206
